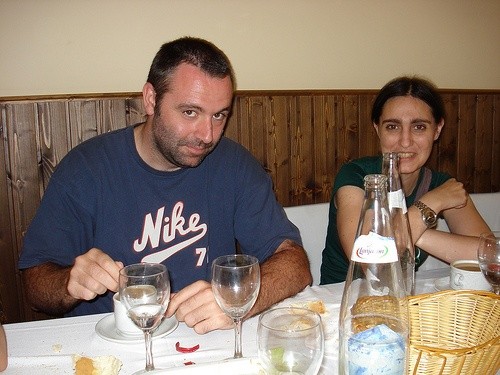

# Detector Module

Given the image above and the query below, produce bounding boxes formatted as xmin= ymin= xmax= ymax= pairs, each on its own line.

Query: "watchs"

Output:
xmin=414 ymin=200 xmax=438 ymax=228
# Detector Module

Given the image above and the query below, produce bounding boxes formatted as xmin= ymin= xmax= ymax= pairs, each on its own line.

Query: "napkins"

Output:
xmin=145 ymin=356 xmax=254 ymax=375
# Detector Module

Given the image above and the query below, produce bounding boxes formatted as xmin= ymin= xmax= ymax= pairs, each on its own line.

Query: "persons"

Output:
xmin=17 ymin=36 xmax=313 ymax=335
xmin=318 ymin=75 xmax=497 ymax=285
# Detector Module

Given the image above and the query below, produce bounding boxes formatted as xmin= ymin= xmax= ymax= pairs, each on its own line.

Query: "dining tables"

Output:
xmin=0 ymin=269 xmax=500 ymax=375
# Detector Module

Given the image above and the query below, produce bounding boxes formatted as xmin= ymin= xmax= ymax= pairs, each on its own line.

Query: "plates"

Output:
xmin=432 ymin=276 xmax=450 ymax=292
xmin=95 ymin=313 xmax=178 ymax=343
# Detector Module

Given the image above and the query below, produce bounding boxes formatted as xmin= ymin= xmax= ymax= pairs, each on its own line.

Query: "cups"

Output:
xmin=112 ymin=285 xmax=157 ymax=334
xmin=451 ymin=260 xmax=492 ymax=292
xmin=256 ymin=307 xmax=324 ymax=375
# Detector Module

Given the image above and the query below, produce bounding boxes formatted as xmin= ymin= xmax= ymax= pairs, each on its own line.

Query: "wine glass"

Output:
xmin=210 ymin=254 xmax=261 ymax=359
xmin=118 ymin=262 xmax=170 ymax=375
xmin=477 ymin=231 xmax=500 ymax=296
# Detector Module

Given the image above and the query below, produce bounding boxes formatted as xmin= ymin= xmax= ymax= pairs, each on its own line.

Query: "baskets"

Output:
xmin=351 ymin=289 xmax=500 ymax=375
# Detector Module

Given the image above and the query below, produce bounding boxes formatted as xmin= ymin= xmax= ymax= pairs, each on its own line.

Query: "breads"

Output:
xmin=72 ymin=355 xmax=100 ymax=375
xmin=308 ymin=299 xmax=326 ymax=313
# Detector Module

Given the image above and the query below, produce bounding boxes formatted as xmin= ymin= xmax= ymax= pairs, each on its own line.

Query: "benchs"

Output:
xmin=283 ymin=188 xmax=500 ymax=291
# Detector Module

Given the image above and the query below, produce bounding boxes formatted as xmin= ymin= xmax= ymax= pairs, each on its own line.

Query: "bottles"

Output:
xmin=382 ymin=152 xmax=416 ymax=296
xmin=338 ymin=174 xmax=409 ymax=375
